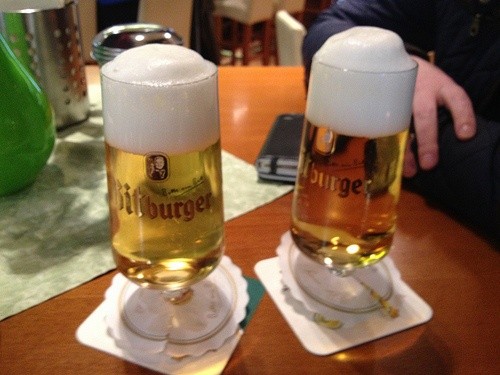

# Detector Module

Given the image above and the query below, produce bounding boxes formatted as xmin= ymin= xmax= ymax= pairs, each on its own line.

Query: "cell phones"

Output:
xmin=256 ymin=113 xmax=305 ymax=184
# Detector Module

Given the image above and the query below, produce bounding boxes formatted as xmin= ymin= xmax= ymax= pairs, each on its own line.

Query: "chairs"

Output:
xmin=137 ymin=0 xmax=204 ymax=56
xmin=212 ymin=0 xmax=308 ymax=66
xmin=276 ymin=9 xmax=304 ymax=67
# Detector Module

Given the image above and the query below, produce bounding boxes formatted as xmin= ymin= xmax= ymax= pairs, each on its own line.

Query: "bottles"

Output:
xmin=0 ymin=31 xmax=58 ymax=196
xmin=91 ymin=24 xmax=185 ymax=69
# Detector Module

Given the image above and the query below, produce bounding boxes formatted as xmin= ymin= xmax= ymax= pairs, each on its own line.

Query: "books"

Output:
xmin=256 ymin=114 xmax=304 ymax=183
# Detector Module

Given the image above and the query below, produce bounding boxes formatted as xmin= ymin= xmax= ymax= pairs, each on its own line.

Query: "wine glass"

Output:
xmin=289 ymin=47 xmax=419 ymax=313
xmin=100 ymin=56 xmax=238 ymax=345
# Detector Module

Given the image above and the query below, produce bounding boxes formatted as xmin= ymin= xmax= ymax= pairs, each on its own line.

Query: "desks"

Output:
xmin=0 ymin=68 xmax=500 ymax=375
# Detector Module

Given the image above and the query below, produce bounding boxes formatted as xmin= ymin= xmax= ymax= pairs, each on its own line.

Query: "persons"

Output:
xmin=307 ymin=0 xmax=500 ymax=248
